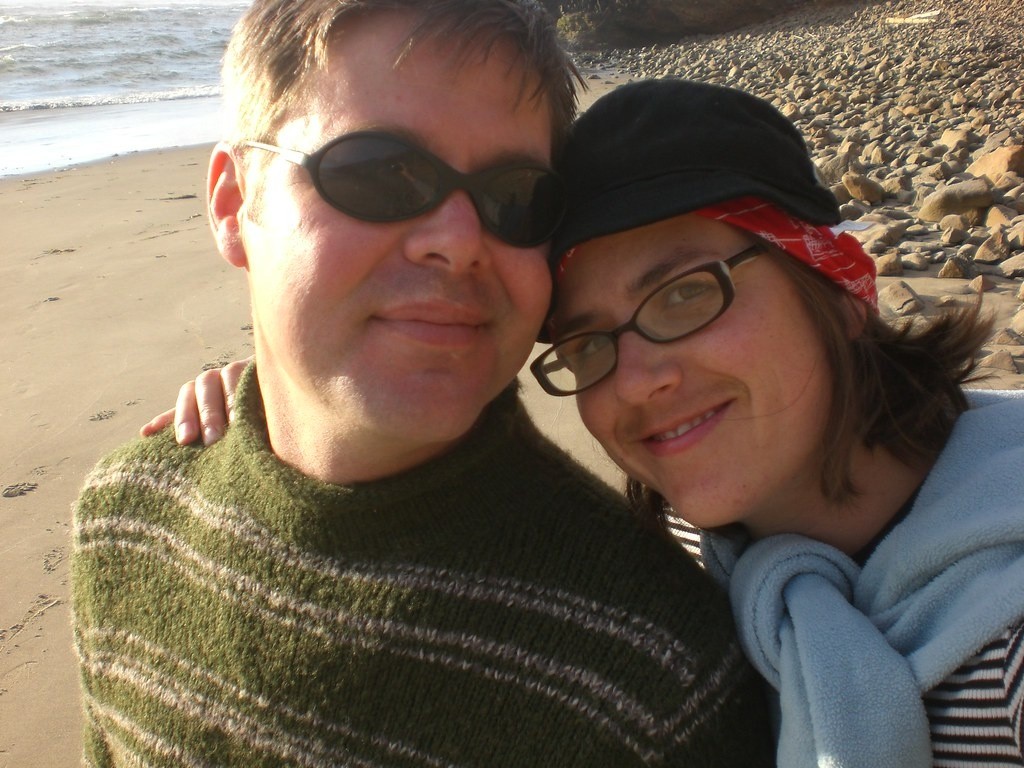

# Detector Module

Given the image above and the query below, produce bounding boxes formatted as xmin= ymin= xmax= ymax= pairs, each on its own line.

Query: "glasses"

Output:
xmin=528 ymin=246 xmax=792 ymax=397
xmin=228 ymin=134 xmax=566 ymax=248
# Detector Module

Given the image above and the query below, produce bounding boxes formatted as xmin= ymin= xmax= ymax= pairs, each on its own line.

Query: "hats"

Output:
xmin=537 ymin=75 xmax=842 ymax=333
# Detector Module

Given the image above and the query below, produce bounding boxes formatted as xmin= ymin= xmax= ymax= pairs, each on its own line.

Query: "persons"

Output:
xmin=70 ymin=0 xmax=778 ymax=767
xmin=137 ymin=78 xmax=1024 ymax=768
